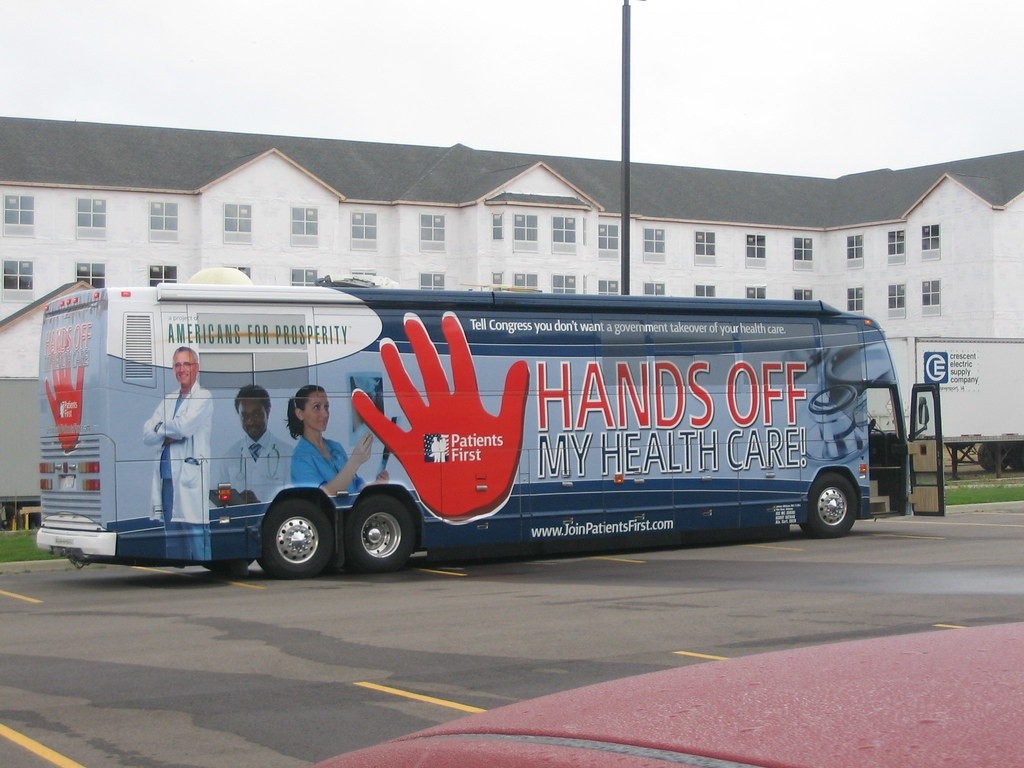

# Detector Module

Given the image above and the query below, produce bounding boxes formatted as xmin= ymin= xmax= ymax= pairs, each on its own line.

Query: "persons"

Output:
xmin=286 ymin=384 xmax=390 ymax=507
xmin=143 ymin=347 xmax=213 ymax=561
xmin=218 ymin=384 xmax=294 ymax=504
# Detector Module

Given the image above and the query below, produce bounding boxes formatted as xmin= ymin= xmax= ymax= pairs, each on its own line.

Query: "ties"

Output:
xmin=249 ymin=443 xmax=263 ymax=461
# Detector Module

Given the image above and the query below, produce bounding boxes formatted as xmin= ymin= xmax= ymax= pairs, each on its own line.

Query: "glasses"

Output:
xmin=174 ymin=362 xmax=199 ymax=370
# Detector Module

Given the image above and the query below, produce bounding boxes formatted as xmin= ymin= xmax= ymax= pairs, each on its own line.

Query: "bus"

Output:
xmin=35 ymin=265 xmax=946 ymax=582
